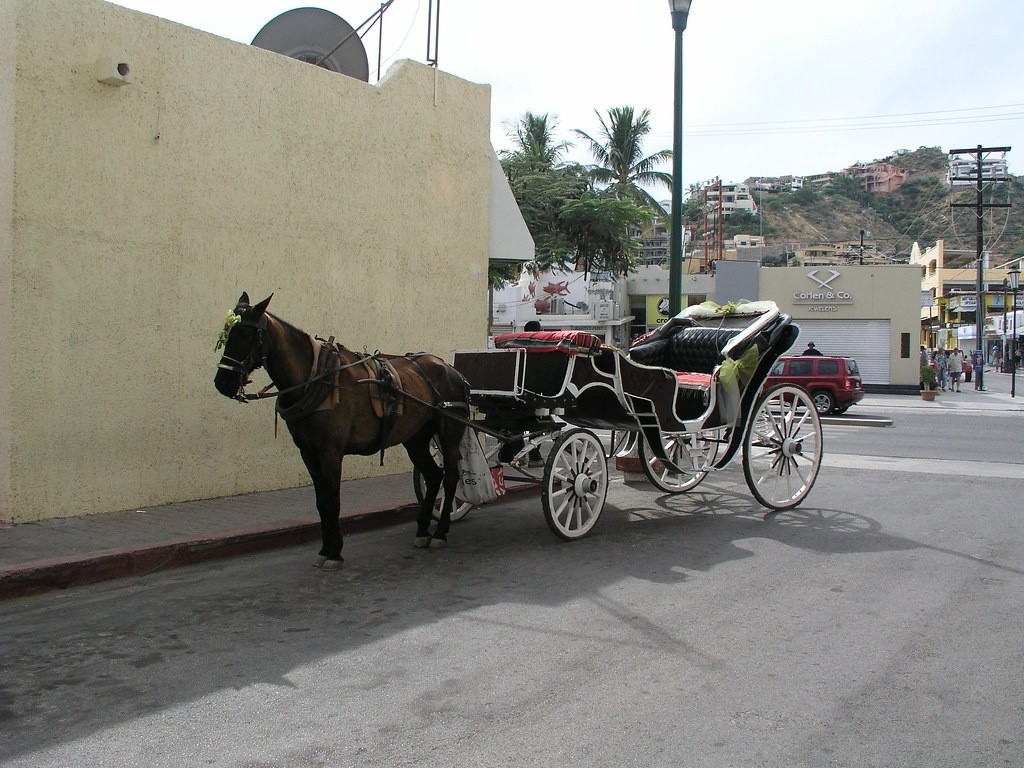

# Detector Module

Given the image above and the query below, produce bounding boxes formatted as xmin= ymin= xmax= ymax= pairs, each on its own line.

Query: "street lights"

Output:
xmin=1007 ymin=265 xmax=1022 ymax=398
xmin=668 ymin=0 xmax=690 ymax=474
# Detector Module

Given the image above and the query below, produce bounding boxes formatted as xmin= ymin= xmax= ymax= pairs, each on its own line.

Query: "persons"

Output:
xmin=517 ymin=321 xmax=542 ymax=467
xmin=945 ymin=350 xmax=952 ymax=390
xmin=991 ymin=346 xmax=999 ymax=372
xmin=920 ymin=346 xmax=929 ymax=367
xmin=947 ymin=348 xmax=964 ymax=392
xmin=934 ymin=348 xmax=947 ymax=391
xmin=802 ymin=342 xmax=823 ymax=356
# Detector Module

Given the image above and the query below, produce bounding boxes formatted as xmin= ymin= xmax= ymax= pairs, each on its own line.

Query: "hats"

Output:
xmin=920 ymin=346 xmax=926 ymax=351
xmin=807 ymin=342 xmax=815 ymax=347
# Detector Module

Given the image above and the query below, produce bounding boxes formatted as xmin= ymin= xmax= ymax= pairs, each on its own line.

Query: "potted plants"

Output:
xmin=919 ymin=366 xmax=937 ymax=402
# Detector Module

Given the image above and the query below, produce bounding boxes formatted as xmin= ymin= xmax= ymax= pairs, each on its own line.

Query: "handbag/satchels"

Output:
xmin=451 ymin=424 xmax=497 ymax=505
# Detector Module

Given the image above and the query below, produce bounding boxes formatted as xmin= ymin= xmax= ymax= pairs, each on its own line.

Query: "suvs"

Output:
xmin=762 ymin=353 xmax=865 ymax=416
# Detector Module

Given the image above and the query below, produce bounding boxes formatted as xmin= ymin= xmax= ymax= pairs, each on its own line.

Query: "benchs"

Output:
xmin=631 ymin=326 xmax=743 ymax=403
xmin=493 ymin=331 xmax=600 ymax=357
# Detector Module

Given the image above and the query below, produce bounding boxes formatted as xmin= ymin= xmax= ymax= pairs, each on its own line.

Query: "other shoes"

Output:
xmin=528 ymin=456 xmax=546 ymax=469
xmin=950 ymin=389 xmax=954 ymax=392
xmin=942 ymin=389 xmax=946 ymax=392
xmin=956 ymin=390 xmax=961 ymax=392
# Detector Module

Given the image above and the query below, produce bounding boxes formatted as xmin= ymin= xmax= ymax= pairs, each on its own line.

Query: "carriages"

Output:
xmin=211 ymin=290 xmax=825 ymax=573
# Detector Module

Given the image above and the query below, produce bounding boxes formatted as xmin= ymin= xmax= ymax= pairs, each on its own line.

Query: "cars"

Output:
xmin=932 ymin=349 xmax=972 ymax=384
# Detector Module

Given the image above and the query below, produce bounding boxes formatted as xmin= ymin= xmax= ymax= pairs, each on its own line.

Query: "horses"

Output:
xmin=213 ymin=292 xmax=470 ymax=571
